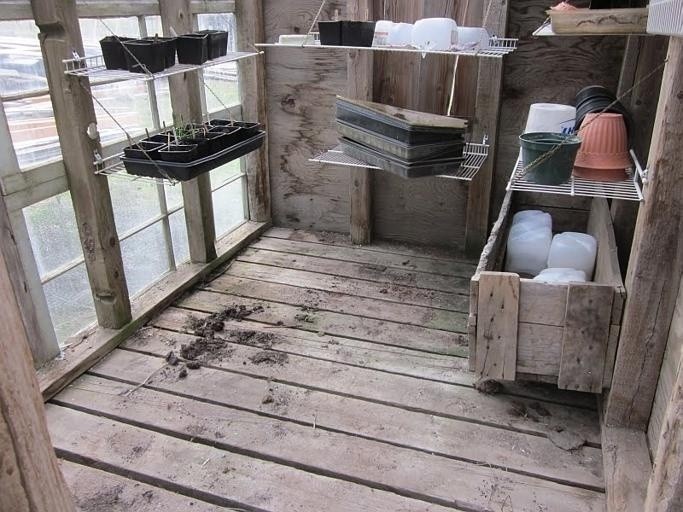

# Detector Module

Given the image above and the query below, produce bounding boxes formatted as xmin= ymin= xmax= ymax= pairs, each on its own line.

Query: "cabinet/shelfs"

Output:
xmin=254 ymin=32 xmax=519 ymax=182
xmin=506 ymin=22 xmax=657 ymax=202
xmin=61 ymin=51 xmax=263 ymax=186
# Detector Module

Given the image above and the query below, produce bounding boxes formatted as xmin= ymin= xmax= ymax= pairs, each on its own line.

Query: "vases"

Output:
xmin=123 ymin=111 xmax=261 ymax=163
xmin=519 ymin=85 xmax=632 ymax=184
xmin=318 ymin=19 xmax=376 ymax=46
xmin=99 ymin=29 xmax=228 ymax=73
